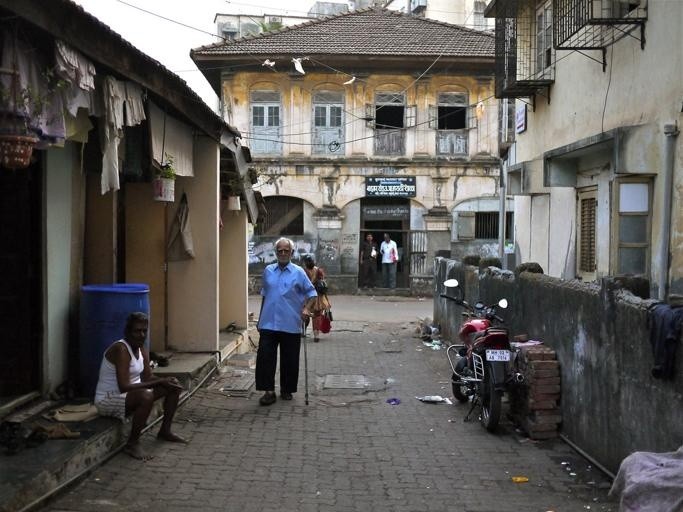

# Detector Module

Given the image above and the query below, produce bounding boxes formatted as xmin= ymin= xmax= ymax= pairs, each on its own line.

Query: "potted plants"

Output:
xmin=0 ymin=63 xmax=75 ymax=169
xmin=152 ymin=151 xmax=176 ymax=202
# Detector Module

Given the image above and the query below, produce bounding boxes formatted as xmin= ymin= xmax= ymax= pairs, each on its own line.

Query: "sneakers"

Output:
xmin=280 ymin=390 xmax=292 ymax=400
xmin=259 ymin=392 xmax=277 ymax=406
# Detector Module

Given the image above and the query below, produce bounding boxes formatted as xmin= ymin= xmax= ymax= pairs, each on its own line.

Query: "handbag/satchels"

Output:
xmin=313 ymin=307 xmax=333 ymax=333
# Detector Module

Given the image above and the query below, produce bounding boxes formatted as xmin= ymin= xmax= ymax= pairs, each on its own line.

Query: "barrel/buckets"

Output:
xmin=78 ymin=281 xmax=152 ymax=387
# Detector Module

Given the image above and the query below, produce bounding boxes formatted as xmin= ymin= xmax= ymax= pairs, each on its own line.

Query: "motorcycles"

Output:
xmin=438 ymin=278 xmax=525 ymax=428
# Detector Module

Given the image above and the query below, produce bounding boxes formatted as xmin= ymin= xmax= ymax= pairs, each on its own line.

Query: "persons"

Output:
xmin=95 ymin=312 xmax=185 ymax=460
xmin=301 ymin=254 xmax=325 ymax=341
xmin=379 ymin=233 xmax=399 ymax=288
xmin=360 ymin=234 xmax=380 ymax=289
xmin=255 ymin=237 xmax=319 ymax=406
